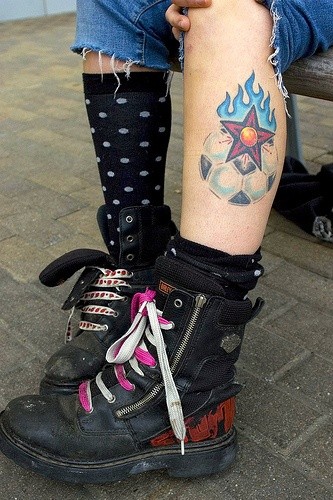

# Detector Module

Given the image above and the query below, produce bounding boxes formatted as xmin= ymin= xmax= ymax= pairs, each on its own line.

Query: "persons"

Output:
xmin=0 ymin=1 xmax=333 ymax=485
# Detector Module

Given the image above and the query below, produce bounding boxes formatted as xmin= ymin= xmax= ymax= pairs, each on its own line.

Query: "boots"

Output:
xmin=0 ymin=253 xmax=265 ymax=483
xmin=40 ymin=202 xmax=171 ymax=391
